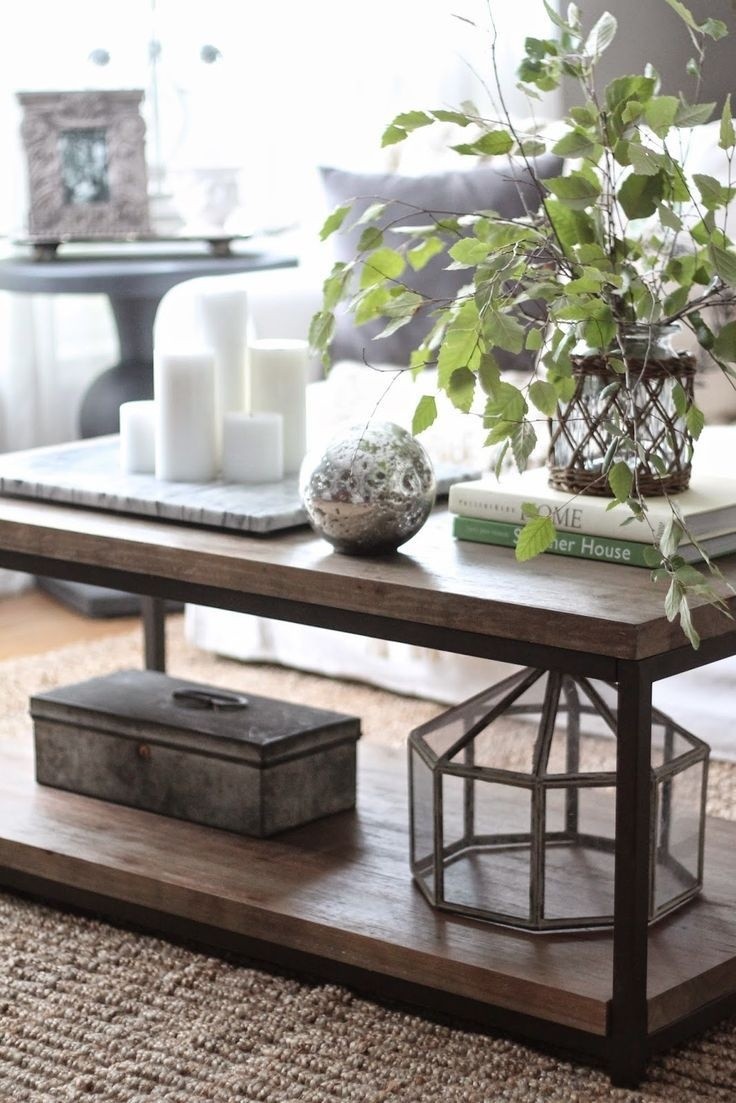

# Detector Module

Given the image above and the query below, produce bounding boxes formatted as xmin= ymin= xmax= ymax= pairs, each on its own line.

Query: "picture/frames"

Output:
xmin=18 ymin=91 xmax=152 ymax=238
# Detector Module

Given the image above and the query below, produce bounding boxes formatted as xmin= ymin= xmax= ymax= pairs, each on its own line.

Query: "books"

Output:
xmin=51 ymin=240 xmax=235 ymax=260
xmin=449 ymin=465 xmax=736 ymax=569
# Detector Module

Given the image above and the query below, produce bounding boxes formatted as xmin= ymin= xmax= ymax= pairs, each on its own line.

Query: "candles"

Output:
xmin=118 ymin=337 xmax=310 ymax=486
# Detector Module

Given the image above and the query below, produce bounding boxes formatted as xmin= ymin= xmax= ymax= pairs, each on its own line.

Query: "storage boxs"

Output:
xmin=30 ymin=667 xmax=363 ymax=837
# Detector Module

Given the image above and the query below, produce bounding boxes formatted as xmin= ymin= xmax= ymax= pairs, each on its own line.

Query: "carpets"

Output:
xmin=0 ymin=618 xmax=736 ymax=1103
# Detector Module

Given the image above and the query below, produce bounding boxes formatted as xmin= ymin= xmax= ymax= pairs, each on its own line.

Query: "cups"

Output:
xmin=176 ymin=171 xmax=236 ymax=232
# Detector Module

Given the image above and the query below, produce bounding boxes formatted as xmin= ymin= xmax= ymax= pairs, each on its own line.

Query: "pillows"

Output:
xmin=315 ymin=154 xmax=566 ymax=373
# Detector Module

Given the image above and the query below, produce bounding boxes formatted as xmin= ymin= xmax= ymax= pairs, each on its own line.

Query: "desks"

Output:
xmin=0 ymin=252 xmax=300 ymax=616
xmin=0 ymin=489 xmax=736 ymax=1089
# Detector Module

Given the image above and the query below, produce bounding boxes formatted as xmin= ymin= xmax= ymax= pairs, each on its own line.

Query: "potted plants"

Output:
xmin=309 ymin=0 xmax=736 ymax=650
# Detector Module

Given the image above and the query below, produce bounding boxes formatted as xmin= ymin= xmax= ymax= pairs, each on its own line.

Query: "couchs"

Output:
xmin=153 ymin=265 xmax=736 ymax=760
